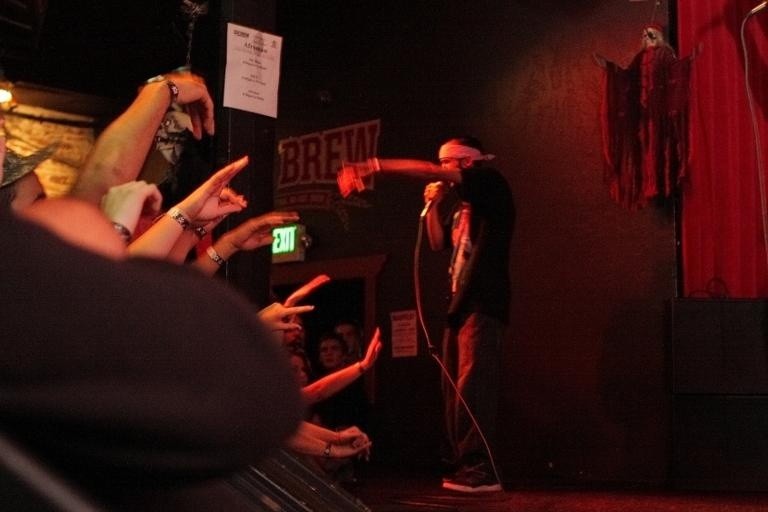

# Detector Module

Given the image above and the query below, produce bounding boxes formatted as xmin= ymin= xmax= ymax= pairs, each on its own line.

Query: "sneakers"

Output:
xmin=442 ymin=467 xmax=502 ymax=493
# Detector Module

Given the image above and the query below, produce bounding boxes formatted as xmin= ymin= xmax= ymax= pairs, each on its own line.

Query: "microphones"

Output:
xmin=420 ymin=181 xmax=444 ymax=218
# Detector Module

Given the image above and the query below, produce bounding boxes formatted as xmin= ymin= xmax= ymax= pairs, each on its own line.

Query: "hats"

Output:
xmin=1 ymin=140 xmax=61 ymax=187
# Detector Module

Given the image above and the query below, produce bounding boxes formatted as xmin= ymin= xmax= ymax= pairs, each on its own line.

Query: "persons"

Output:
xmin=1 ymin=69 xmax=385 ymax=512
xmin=344 ymin=140 xmax=514 ymax=494
